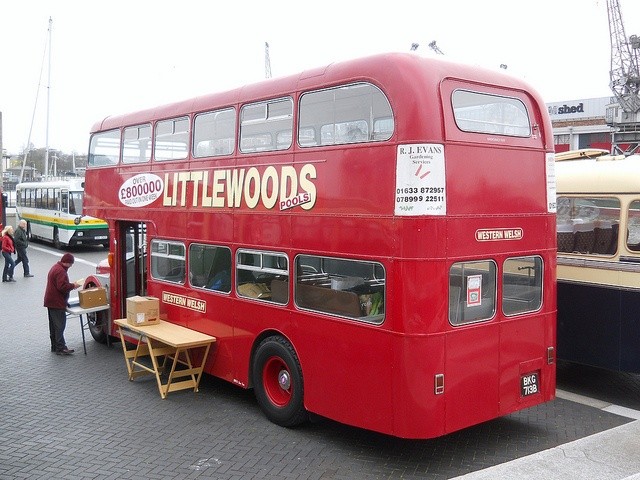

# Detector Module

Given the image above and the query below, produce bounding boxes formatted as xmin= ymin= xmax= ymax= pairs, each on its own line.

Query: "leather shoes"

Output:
xmin=25 ymin=274 xmax=34 ymax=277
xmin=57 ymin=349 xmax=74 ymax=354
xmin=9 ymin=278 xmax=16 ymax=282
xmin=3 ymin=279 xmax=8 ymax=282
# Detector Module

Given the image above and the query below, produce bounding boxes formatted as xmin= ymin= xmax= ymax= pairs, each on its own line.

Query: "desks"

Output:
xmin=62 ymin=298 xmax=109 ymax=358
xmin=113 ymin=319 xmax=216 ymax=400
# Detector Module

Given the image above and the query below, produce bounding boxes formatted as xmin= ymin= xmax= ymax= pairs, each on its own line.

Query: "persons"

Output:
xmin=1 ymin=226 xmax=16 ymax=282
xmin=7 ymin=219 xmax=34 ymax=277
xmin=43 ymin=253 xmax=82 ymax=354
xmin=0 ymin=185 xmax=8 ymax=229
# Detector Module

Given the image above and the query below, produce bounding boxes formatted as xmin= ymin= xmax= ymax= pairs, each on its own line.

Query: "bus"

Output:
xmin=83 ymin=53 xmax=558 ymax=441
xmin=550 ymin=148 xmax=640 ymax=372
xmin=14 ymin=182 xmax=109 ymax=252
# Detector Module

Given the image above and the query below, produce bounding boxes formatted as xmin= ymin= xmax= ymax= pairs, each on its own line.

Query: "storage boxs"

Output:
xmin=126 ymin=295 xmax=159 ymax=327
xmin=77 ymin=286 xmax=107 ymax=309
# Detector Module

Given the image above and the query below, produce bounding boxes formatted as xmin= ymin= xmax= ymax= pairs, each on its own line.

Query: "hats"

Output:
xmin=61 ymin=253 xmax=74 ymax=265
